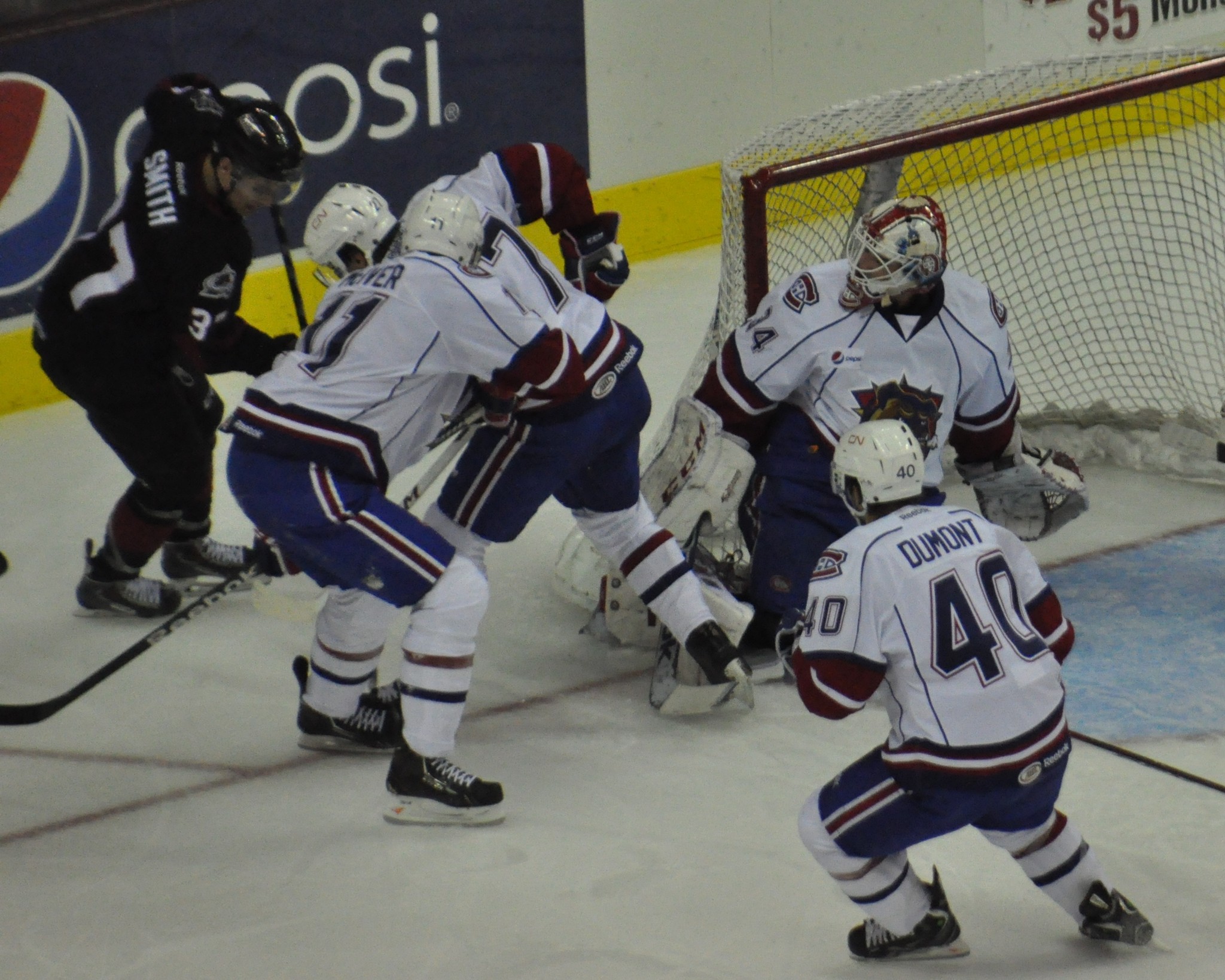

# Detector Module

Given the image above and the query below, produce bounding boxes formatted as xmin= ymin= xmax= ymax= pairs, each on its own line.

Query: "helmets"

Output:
xmin=304 ymin=181 xmax=399 ymax=289
xmin=838 ymin=197 xmax=947 ymax=308
xmin=399 ymin=188 xmax=485 ymax=268
xmin=830 ymin=420 xmax=924 ymax=516
xmin=210 ymin=100 xmax=303 ymax=181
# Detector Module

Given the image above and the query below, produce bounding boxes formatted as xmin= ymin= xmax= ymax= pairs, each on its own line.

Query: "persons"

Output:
xmin=774 ymin=419 xmax=1169 ymax=960
xmin=33 ymin=74 xmax=304 ymax=618
xmin=301 ymin=142 xmax=755 ymax=734
xmin=217 ymin=190 xmax=594 ymax=826
xmin=605 ymin=194 xmax=1086 ymax=717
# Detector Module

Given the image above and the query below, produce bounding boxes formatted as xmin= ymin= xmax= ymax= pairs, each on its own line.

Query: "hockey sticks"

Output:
xmin=398 ymin=257 xmax=616 ymax=514
xmin=268 ymin=207 xmax=311 ymax=336
xmin=0 ymin=557 xmax=265 ymax=727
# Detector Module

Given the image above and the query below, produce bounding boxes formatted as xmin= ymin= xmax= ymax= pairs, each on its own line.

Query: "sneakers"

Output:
xmin=161 ymin=535 xmax=256 ymax=591
xmin=292 ymin=656 xmax=402 ymax=754
xmin=847 ymin=864 xmax=971 ymax=961
xmin=381 ymin=739 xmax=506 ymax=824
xmin=685 ymin=620 xmax=754 ymax=709
xmin=1078 ymin=880 xmax=1156 ymax=947
xmin=73 ymin=538 xmax=182 ymax=618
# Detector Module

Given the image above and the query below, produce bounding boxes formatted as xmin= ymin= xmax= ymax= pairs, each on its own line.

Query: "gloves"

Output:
xmin=559 ymin=210 xmax=631 ymax=302
xmin=476 ymin=377 xmax=515 ymax=427
xmin=775 ymin=607 xmax=805 ymax=679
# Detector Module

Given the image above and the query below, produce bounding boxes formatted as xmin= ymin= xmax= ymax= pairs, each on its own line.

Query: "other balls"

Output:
xmin=1 ymin=551 xmax=14 ymax=578
